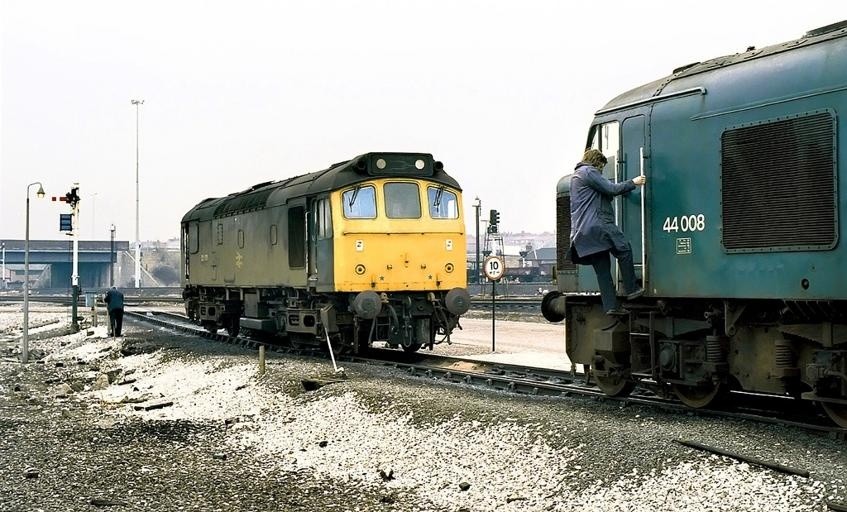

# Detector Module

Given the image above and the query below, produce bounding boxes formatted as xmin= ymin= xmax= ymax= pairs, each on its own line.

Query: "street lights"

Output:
xmin=22 ymin=182 xmax=45 ymax=365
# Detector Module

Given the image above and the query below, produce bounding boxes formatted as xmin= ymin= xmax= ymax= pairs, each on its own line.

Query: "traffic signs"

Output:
xmin=484 ymin=257 xmax=505 ymax=281
xmin=60 ymin=214 xmax=71 ymax=231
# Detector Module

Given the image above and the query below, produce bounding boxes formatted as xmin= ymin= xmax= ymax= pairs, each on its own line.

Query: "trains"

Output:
xmin=540 ymin=18 xmax=847 ymax=434
xmin=180 ymin=152 xmax=471 ymax=356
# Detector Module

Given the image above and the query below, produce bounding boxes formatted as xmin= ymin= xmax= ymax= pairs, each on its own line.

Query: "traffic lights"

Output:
xmin=490 ymin=209 xmax=500 ymax=225
xmin=492 ymin=226 xmax=497 ymax=233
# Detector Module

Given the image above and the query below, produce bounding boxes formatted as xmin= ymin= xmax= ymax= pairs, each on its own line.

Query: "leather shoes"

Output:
xmin=627 ymin=287 xmax=647 ymax=300
xmin=605 ymin=306 xmax=632 ymax=316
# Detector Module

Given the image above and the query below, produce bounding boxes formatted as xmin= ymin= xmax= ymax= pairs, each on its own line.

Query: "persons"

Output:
xmin=564 ymin=147 xmax=647 ymax=316
xmin=103 ymin=285 xmax=126 ymax=337
